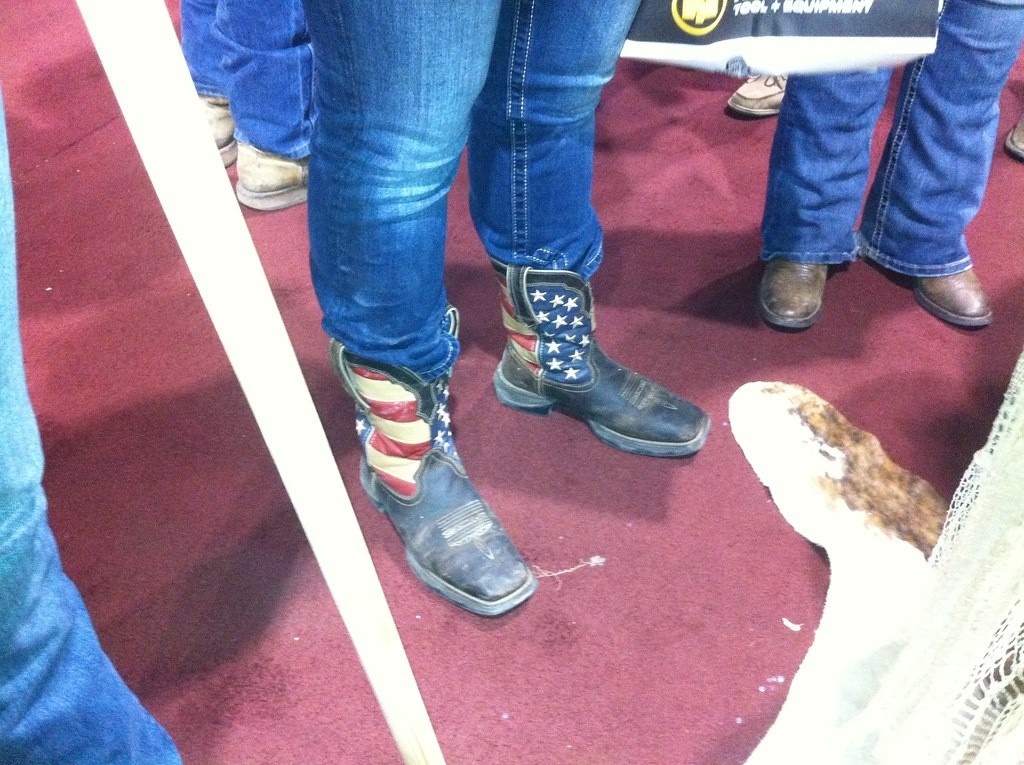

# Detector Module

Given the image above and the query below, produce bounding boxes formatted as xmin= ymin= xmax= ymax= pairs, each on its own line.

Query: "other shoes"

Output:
xmin=199 ymin=96 xmax=238 ymax=169
xmin=728 ymin=74 xmax=787 ymax=116
xmin=1004 ymin=113 xmax=1024 ymax=159
xmin=235 ymin=143 xmax=309 ymax=210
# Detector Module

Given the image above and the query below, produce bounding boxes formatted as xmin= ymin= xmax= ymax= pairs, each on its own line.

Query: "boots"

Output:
xmin=327 ymin=304 xmax=536 ymax=618
xmin=491 ymin=257 xmax=712 ymax=457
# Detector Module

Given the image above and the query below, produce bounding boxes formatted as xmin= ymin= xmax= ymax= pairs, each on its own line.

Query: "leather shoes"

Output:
xmin=756 ymin=259 xmax=830 ymax=327
xmin=915 ymin=268 xmax=994 ymax=327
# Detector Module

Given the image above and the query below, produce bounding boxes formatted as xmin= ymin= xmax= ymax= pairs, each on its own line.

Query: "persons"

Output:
xmin=181 ymin=0 xmax=313 ymax=211
xmin=727 ymin=1 xmax=1023 ymax=330
xmin=303 ymin=0 xmax=710 ymax=616
xmin=0 ymin=102 xmax=185 ymax=765
